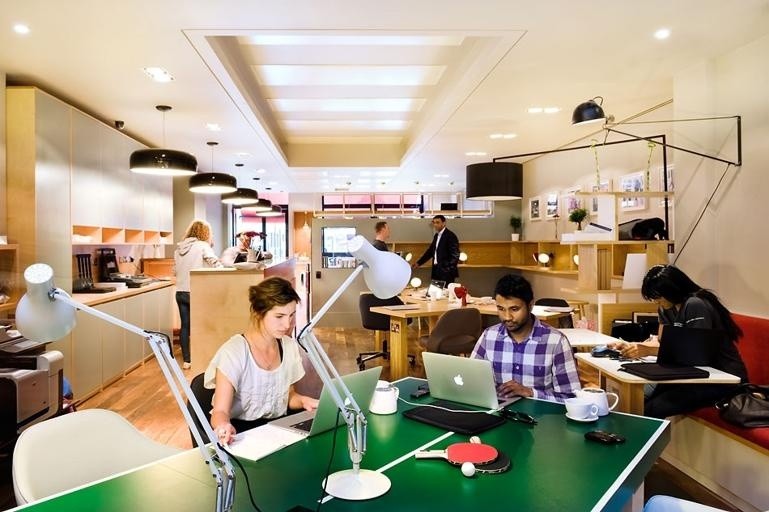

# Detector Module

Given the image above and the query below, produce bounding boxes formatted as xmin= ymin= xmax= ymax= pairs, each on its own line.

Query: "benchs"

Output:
xmin=597 ymin=290 xmax=769 ymax=512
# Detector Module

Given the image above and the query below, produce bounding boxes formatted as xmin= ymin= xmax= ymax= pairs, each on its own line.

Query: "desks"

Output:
xmin=553 ymin=325 xmax=623 ymax=352
xmin=575 ymin=342 xmax=745 ymax=423
xmin=0 ymin=364 xmax=682 ymax=512
xmin=365 ymin=291 xmax=576 ymax=383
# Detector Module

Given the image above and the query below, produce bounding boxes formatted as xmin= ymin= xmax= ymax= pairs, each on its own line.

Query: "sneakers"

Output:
xmin=183 ymin=360 xmax=191 ymax=369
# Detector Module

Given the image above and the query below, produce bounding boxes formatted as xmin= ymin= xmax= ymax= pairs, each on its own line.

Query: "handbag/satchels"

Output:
xmin=715 ymin=383 xmax=769 ymax=429
xmin=232 ymin=261 xmax=266 ymax=270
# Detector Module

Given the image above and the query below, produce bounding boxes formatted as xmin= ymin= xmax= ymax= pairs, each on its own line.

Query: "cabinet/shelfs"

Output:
xmin=141 ymin=285 xmax=183 ymax=368
xmin=140 ymin=258 xmax=175 ymax=278
xmin=507 ymin=241 xmax=666 ymax=290
xmin=4 ymin=86 xmax=75 ymax=298
xmin=44 ymin=304 xmax=103 ymax=413
xmin=0 ymin=244 xmax=21 ymax=314
xmin=376 ymin=238 xmax=512 ymax=268
xmin=121 ymin=134 xmax=173 ymax=246
xmin=72 ymin=108 xmax=124 ymax=245
xmin=102 ymin=292 xmax=147 ymax=386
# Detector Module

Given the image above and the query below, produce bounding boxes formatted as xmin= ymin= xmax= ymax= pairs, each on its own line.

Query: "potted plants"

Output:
xmin=507 ymin=214 xmax=524 ymax=242
xmin=566 ymin=204 xmax=593 ymax=232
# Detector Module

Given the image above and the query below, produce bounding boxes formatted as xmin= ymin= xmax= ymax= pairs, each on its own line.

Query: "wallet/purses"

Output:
xmin=584 ymin=430 xmax=624 ymax=445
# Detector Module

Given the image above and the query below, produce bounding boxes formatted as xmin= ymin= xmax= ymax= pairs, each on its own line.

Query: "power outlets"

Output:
xmin=128 ymin=256 xmax=135 ymax=263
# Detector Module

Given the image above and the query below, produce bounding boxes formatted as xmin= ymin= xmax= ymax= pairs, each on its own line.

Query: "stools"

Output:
xmin=568 ymin=299 xmax=592 ymax=326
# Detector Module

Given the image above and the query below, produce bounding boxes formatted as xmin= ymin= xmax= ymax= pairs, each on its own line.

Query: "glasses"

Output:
xmin=499 ymin=408 xmax=538 ymax=424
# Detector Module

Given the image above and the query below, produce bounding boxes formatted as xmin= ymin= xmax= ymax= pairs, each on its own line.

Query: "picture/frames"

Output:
xmin=528 ymin=164 xmax=673 ymax=222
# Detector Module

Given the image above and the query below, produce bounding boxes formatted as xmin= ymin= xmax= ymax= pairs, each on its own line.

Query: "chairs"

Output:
xmin=8 ymin=403 xmax=180 ymax=512
xmin=425 ymin=306 xmax=484 ymax=357
xmin=535 ymin=296 xmax=575 ymax=329
xmin=187 ymin=364 xmax=222 ymax=449
xmin=355 ymin=290 xmax=416 ymax=372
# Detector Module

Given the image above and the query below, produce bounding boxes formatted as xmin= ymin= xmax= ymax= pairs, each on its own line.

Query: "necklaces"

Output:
xmin=258 ymin=348 xmax=278 ymax=369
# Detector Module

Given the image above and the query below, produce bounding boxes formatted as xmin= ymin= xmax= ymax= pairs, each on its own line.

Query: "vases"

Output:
xmin=246 ymin=249 xmax=261 ymax=263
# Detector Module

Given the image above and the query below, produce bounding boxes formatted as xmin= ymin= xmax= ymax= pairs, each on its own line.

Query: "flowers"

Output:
xmin=234 ymin=230 xmax=268 ymax=249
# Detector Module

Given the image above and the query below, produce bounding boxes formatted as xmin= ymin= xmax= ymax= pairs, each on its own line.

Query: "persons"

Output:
xmin=174 ymin=221 xmax=217 ymax=369
xmin=222 ymin=233 xmax=272 ymax=266
xmin=202 ymin=277 xmax=319 ymax=445
xmin=467 ymin=274 xmax=582 ymax=404
xmin=412 ymin=215 xmax=459 ymax=287
xmin=607 ymin=264 xmax=748 ymax=418
xmin=372 ymin=222 xmax=397 ymax=248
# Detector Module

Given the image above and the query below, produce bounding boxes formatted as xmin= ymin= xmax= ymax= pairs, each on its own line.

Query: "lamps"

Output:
xmin=295 ymin=232 xmax=421 ymax=502
xmin=569 ymin=93 xmax=745 ymax=170
xmin=188 ymin=138 xmax=286 ymax=221
xmin=619 ymin=252 xmax=648 ymax=290
xmin=395 ymin=248 xmax=414 ymax=262
xmin=458 ymin=250 xmax=469 ymax=264
xmin=454 ymin=285 xmax=469 ymax=307
xmin=411 ymin=277 xmax=422 ymax=291
xmin=127 ymin=105 xmax=198 ymax=178
xmin=11 ymin=259 xmax=238 ymax=512
xmin=460 ymin=132 xmax=670 ymax=242
xmin=531 ymin=249 xmax=556 ymax=268
